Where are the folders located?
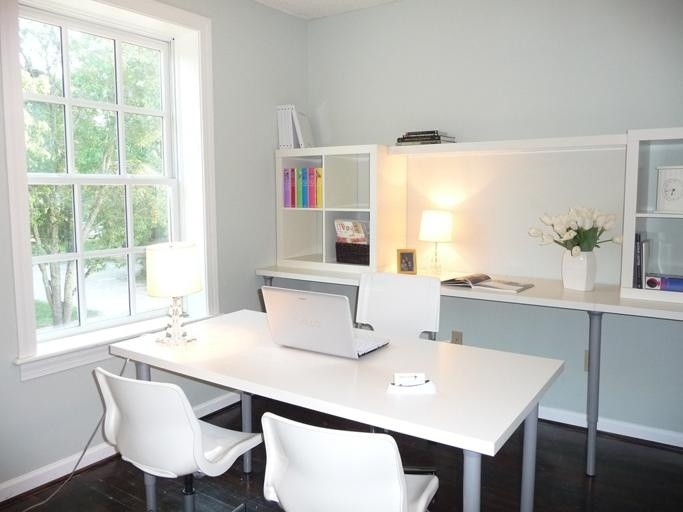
[276,104,316,149]
[282,166,323,208]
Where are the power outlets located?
[451,331,462,344]
[584,350,589,372]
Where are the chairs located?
[260,411,437,511]
[356,270,441,343]
[93,367,261,511]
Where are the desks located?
[110,307,563,512]
[257,267,681,478]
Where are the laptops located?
[260,286,390,359]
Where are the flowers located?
[528,206,624,251]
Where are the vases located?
[561,250,597,292]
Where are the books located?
[633,231,652,291]
[395,129,457,145]
[441,271,536,295]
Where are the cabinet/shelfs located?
[619,129,650,303]
[276,144,405,277]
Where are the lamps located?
[418,209,451,273]
[146,241,206,346]
